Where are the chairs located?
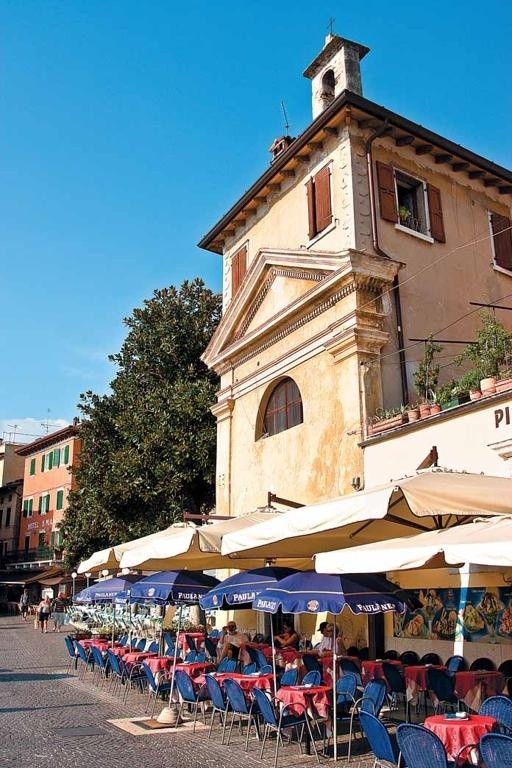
[134,618,143,629]
[111,617,119,628]
[358,647,371,658]
[185,648,196,662]
[84,609,90,620]
[300,654,322,670]
[122,652,158,664]
[137,639,147,652]
[391,721,448,768]
[399,650,419,665]
[425,669,461,714]
[257,663,273,673]
[116,635,127,644]
[279,666,297,686]
[223,678,261,745]
[250,686,325,765]
[149,641,159,653]
[382,649,398,659]
[88,645,106,687]
[88,610,94,620]
[443,656,463,673]
[343,678,386,762]
[105,607,162,621]
[138,664,179,718]
[300,670,322,686]
[420,653,441,666]
[227,658,240,674]
[65,635,91,673]
[250,646,267,667]
[228,641,240,657]
[329,673,361,745]
[64,604,82,616]
[356,709,401,767]
[264,635,274,646]
[106,614,112,626]
[173,669,209,735]
[216,655,228,673]
[86,603,102,609]
[126,620,136,629]
[94,613,99,627]
[165,647,181,658]
[346,643,359,657]
[468,659,495,672]
[129,636,138,647]
[100,616,106,626]
[97,615,102,626]
[74,640,97,683]
[105,649,120,696]
[203,674,241,744]
[186,634,197,650]
[242,662,256,675]
[163,632,175,647]
[383,663,412,717]
[251,633,263,644]
[208,628,218,635]
[360,659,404,687]
[480,734,510,767]
[117,660,147,699]
[205,636,218,657]
[118,618,128,629]
[478,697,512,735]
[195,654,207,662]
[498,660,512,675]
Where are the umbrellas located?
[76,521,203,574]
[118,504,316,572]
[314,522,512,574]
[198,566,299,610]
[251,570,425,614]
[221,465,512,558]
[113,571,222,605]
[72,574,148,604]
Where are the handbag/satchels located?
[34,612,39,630]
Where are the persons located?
[321,623,346,656]
[36,595,52,633]
[19,589,30,620]
[275,622,300,648]
[51,592,67,633]
[316,622,333,656]
[216,621,244,648]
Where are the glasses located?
[229,624,234,629]
[283,624,288,630]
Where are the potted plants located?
[459,369,482,402]
[454,307,512,396]
[435,380,470,410]
[492,360,512,393]
[408,402,419,422]
[414,336,441,418]
[430,391,440,415]
[369,403,405,433]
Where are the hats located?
[318,621,328,633]
